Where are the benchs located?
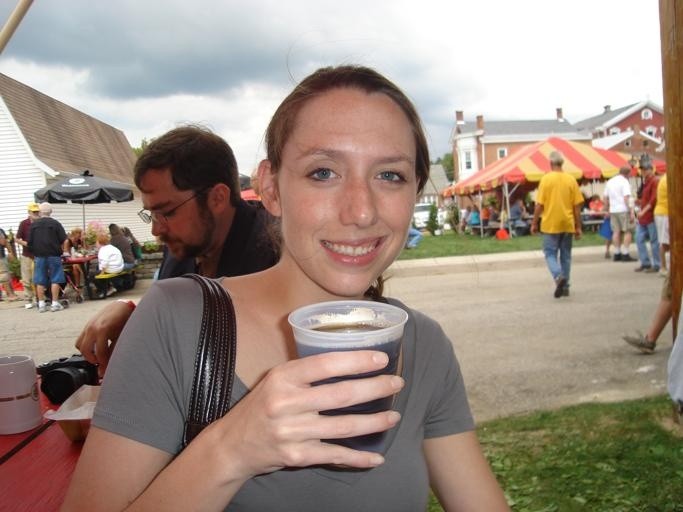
[473,219,527,239]
[94,265,143,298]
[583,219,605,231]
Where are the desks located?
[62,254,97,300]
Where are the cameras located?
[35,350,100,406]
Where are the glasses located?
[138,193,197,225]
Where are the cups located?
[286,298,411,472]
[0,355,43,438]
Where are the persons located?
[621,270,672,353]
[601,165,639,262]
[0,199,145,316]
[597,210,616,260]
[55,63,513,512]
[455,192,646,237]
[528,151,585,298]
[652,172,670,280]
[404,229,423,250]
[71,122,281,377]
[634,161,660,274]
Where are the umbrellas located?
[34,172,134,233]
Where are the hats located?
[638,162,653,170]
[28,204,40,211]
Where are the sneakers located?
[24,303,33,309]
[553,253,670,298]
[39,304,47,313]
[50,303,64,312]
[98,286,117,298]
[622,335,656,354]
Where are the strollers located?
[45,268,84,308]
